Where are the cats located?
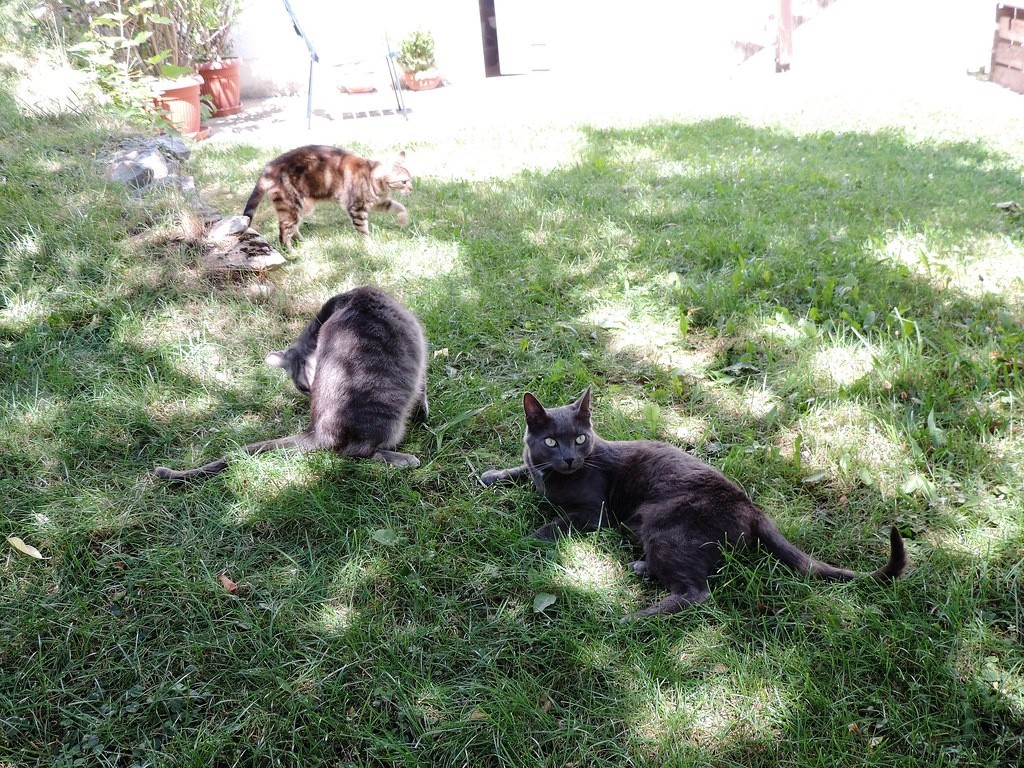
[243,144,413,253]
[480,383,906,624]
[152,286,429,485]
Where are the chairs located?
[284,1,409,129]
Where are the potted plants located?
[395,25,443,91]
[62,0,245,142]
